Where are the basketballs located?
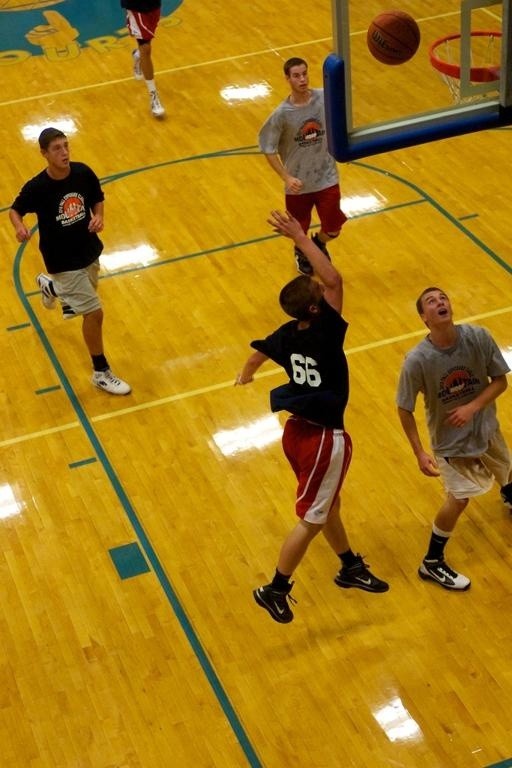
[368,11,419,65]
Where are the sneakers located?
[150,91,164,116]
[92,370,131,395]
[36,273,56,310]
[253,584,293,623]
[418,556,471,591]
[294,232,331,276]
[132,49,143,79]
[335,556,388,593]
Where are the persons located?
[232,211,388,623]
[257,56,348,274]
[395,287,511,593]
[117,0,167,117]
[9,128,132,396]
